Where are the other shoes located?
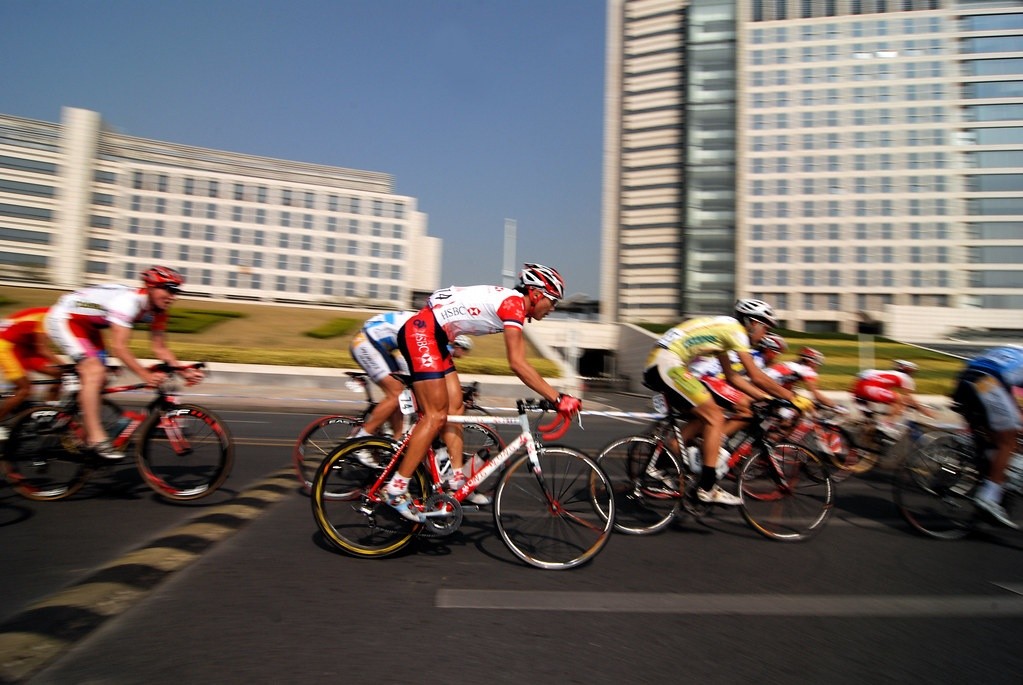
[84,437,125,458]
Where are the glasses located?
[533,286,560,306]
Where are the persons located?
[42,265,203,461]
[643,297,834,506]
[0,305,67,440]
[853,357,936,426]
[380,262,582,521]
[349,311,472,471]
[954,344,1023,531]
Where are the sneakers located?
[450,480,493,505]
[698,485,744,505]
[967,492,1018,527]
[379,488,426,523]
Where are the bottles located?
[715,445,732,470]
[460,448,491,478]
[381,421,396,440]
[109,411,136,439]
[432,440,455,481]
[685,438,702,473]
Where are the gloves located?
[791,394,816,413]
[554,393,583,421]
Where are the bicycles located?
[624,389,945,530]
[0,361,132,469]
[587,380,836,544]
[0,363,237,501]
[293,372,512,508]
[311,372,616,571]
[891,403,1023,541]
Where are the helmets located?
[141,264,183,287]
[734,299,777,329]
[894,358,919,372]
[454,335,472,349]
[798,345,824,367]
[519,261,564,300]
[757,330,788,355]
